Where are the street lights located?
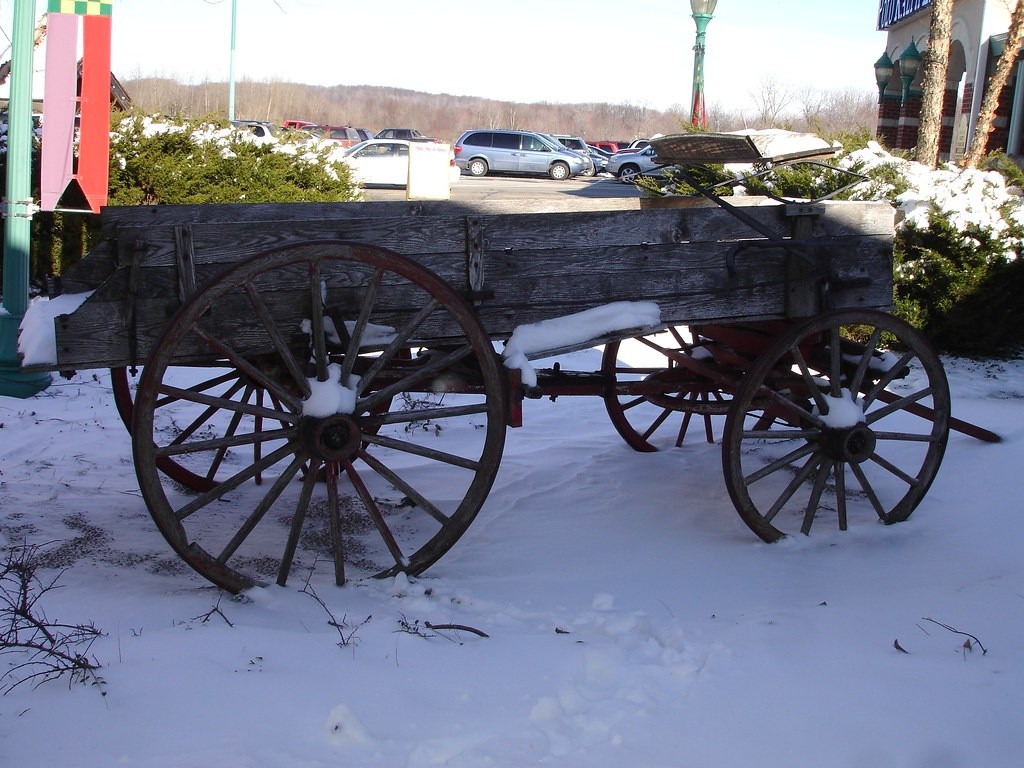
[690,0,717,130]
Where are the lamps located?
[873,45,897,104]
[897,33,925,109]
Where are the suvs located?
[454,128,593,180]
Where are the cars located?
[309,137,460,190]
[531,134,655,178]
[605,145,680,185]
[227,120,441,157]
